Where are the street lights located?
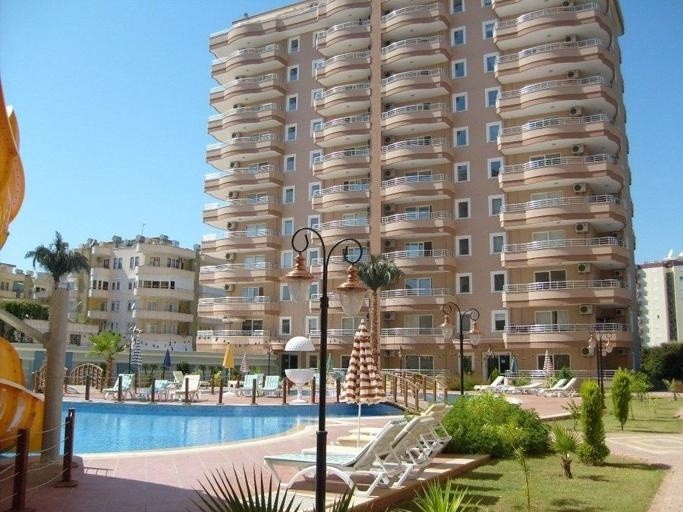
[441,301,481,394]
[588,326,614,394]
[284,226,370,512]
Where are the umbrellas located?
[240,352,250,372]
[130,335,142,393]
[222,341,234,380]
[543,349,555,388]
[511,356,517,373]
[339,319,387,447]
[163,349,171,379]
[326,353,333,372]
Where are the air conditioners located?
[570,142,595,358]
[382,70,397,359]
[222,74,244,292]
[559,2,582,118]
[613,269,626,357]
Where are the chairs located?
[262,402,457,498]
[101,370,284,404]
[472,374,581,399]
